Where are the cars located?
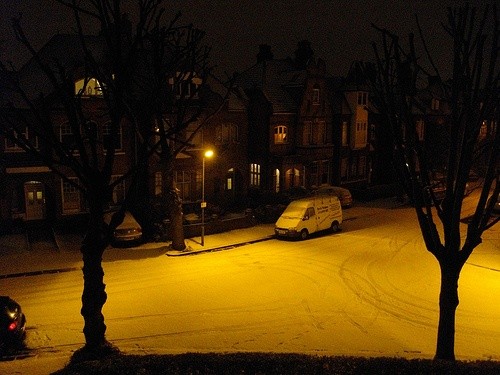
[104,210,144,244]
[317,186,352,207]
[0,295,27,354]
[486,193,500,213]
[406,167,469,206]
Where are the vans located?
[273,193,342,241]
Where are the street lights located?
[201,149,215,247]
